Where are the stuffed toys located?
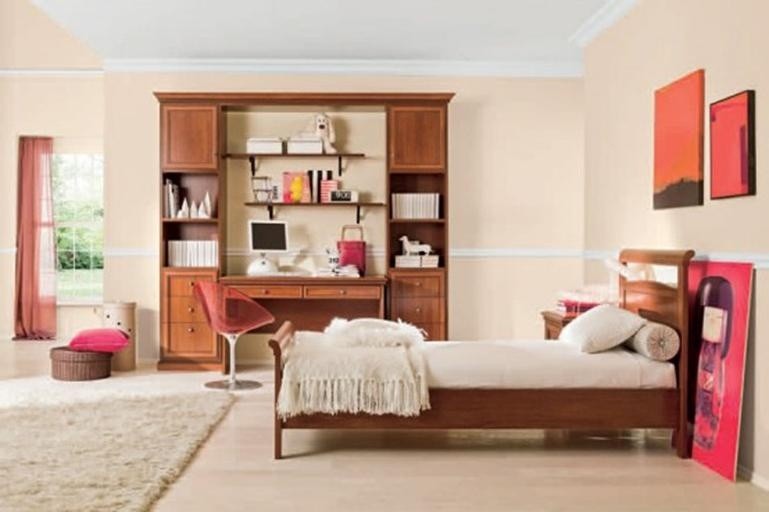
[309,114,339,155]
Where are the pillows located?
[70,328,129,354]
[557,303,647,353]
[622,319,681,361]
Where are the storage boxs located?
[50,347,112,380]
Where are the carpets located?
[1,340,237,512]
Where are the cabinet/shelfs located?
[153,93,456,373]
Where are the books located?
[168,239,218,267]
[395,255,439,266]
[554,298,598,319]
[392,192,440,219]
[164,177,179,219]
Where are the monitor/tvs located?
[248,221,288,274]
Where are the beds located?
[268,248,695,460]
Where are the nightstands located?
[541,309,573,340]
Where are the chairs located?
[191,279,275,393]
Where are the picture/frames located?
[653,69,704,210]
[709,90,756,199]
